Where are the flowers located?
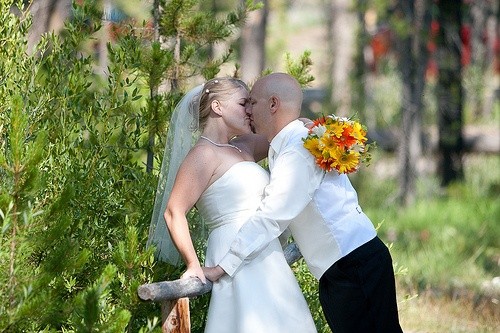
[302,111,376,174]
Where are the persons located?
[163,77,318,332]
[201,72,403,333]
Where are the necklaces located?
[200,135,242,153]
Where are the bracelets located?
[304,122,315,127]
